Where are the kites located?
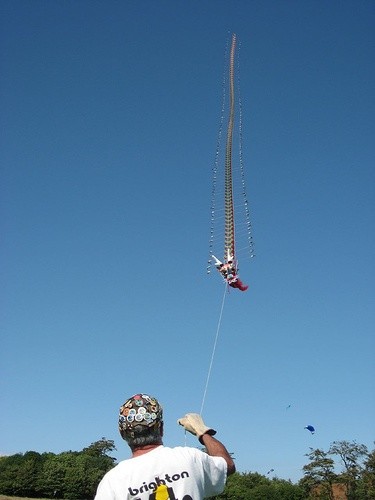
[304,424,316,436]
[206,31,257,291]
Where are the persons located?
[94,393,237,499]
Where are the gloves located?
[179,413,216,444]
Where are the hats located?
[119,393,162,438]
